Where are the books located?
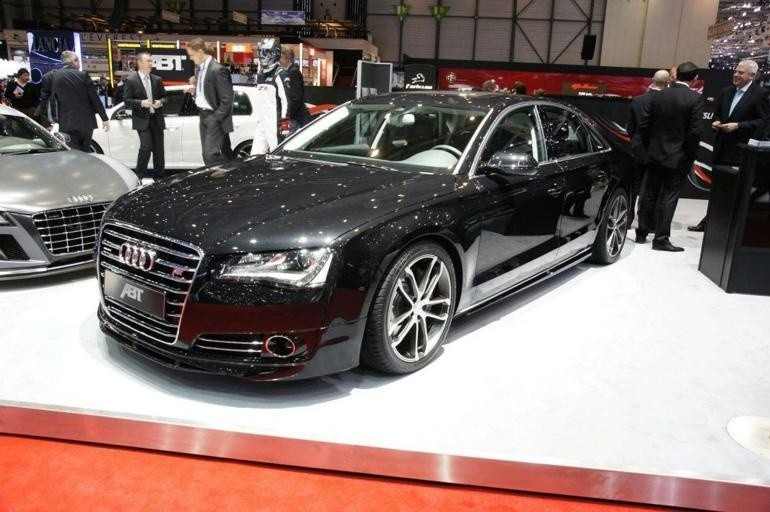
[14,86,24,95]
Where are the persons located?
[627,69,670,233]
[481,79,545,96]
[249,36,291,154]
[635,60,704,252]
[187,38,233,165]
[0,73,126,136]
[687,59,770,232]
[277,46,313,135]
[645,66,678,91]
[123,52,167,185]
[4,68,39,120]
[40,50,111,153]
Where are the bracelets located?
[736,122,738,130]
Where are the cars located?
[47,83,267,171]
[0,104,142,283]
[95,87,634,383]
[279,102,337,135]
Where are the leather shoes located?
[687,221,704,232]
[635,233,646,243]
[652,237,684,252]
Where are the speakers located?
[581,35,596,60]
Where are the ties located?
[199,65,204,94]
[143,76,152,100]
[729,90,743,117]
